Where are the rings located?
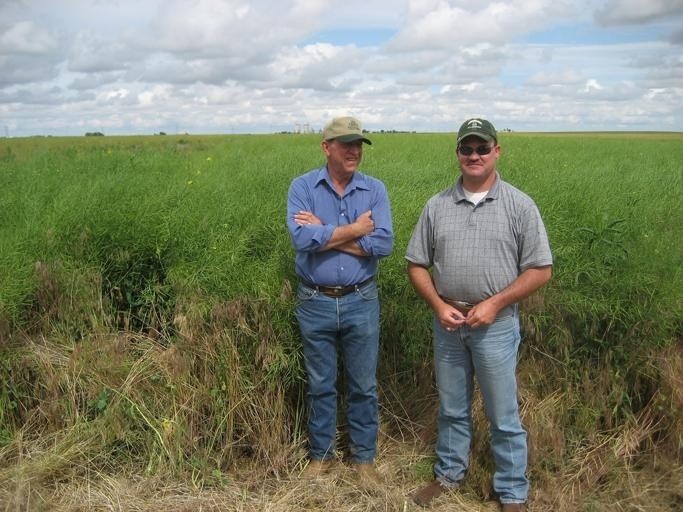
[307,223,311,225]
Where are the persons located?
[284,115,395,485]
[404,117,554,512]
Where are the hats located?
[456,118,498,146]
[323,115,372,148]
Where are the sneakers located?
[352,462,372,481]
[301,458,332,479]
[502,501,527,512]
[414,479,466,507]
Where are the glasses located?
[458,146,496,155]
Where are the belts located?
[302,275,375,297]
[441,297,476,315]
